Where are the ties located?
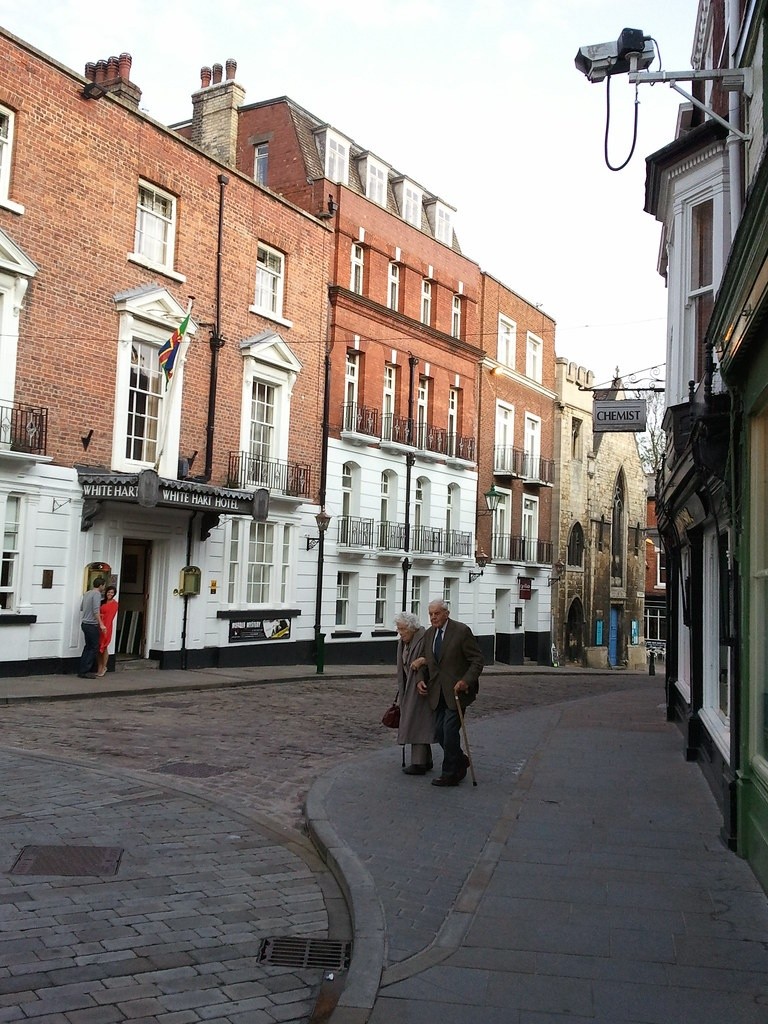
[434,629,443,661]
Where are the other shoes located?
[432,775,458,787]
[403,761,433,776]
[456,754,470,780]
[78,673,96,679]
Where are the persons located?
[93,586,119,676]
[393,611,438,775]
[415,599,485,786]
[78,578,106,679]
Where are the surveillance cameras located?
[574,35,655,82]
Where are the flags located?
[158,312,189,392]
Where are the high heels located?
[95,667,107,676]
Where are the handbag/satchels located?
[382,691,400,729]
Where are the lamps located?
[469,546,489,583]
[547,559,565,587]
[476,481,503,517]
